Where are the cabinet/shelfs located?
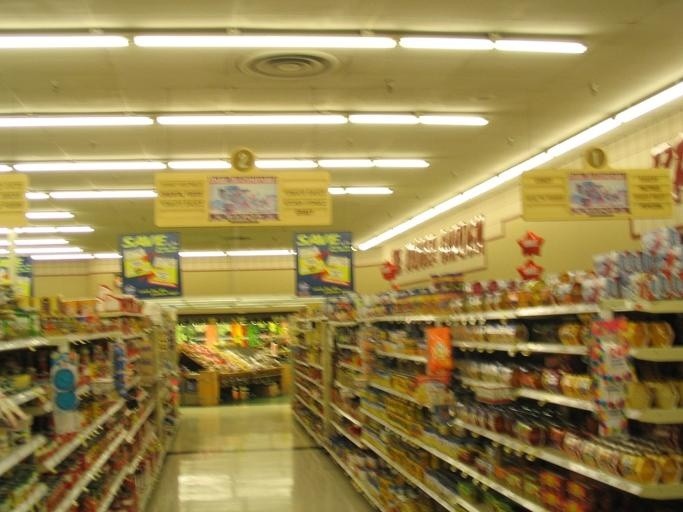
[291,296,683,512]
[0,325,180,512]
